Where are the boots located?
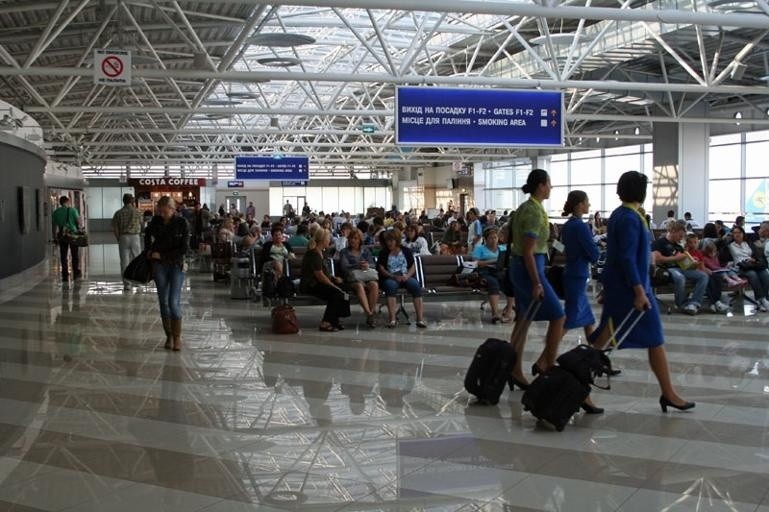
[163,318,174,349]
[172,319,182,351]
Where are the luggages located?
[522,304,649,432]
[464,296,544,404]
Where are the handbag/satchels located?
[57,226,88,247]
[449,272,479,286]
[556,344,617,384]
[348,267,378,282]
[271,305,297,334]
[498,266,515,295]
[546,266,567,300]
[123,250,153,284]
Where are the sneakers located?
[417,320,427,327]
[729,279,747,289]
[712,302,734,313]
[683,304,697,314]
[759,299,769,311]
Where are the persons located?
[262,332,417,427]
[52,197,81,281]
[114,291,142,376]
[113,194,144,290]
[507,390,694,512]
[532,191,624,377]
[144,196,191,351]
[507,170,567,390]
[578,171,695,413]
[138,353,194,482]
[681,311,769,384]
[55,282,86,362]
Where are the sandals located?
[319,324,337,332]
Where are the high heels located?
[508,374,530,391]
[532,363,545,375]
[597,367,621,376]
[660,395,695,413]
[580,401,603,414]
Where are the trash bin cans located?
[230,257,253,299]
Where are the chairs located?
[228,205,763,309]
